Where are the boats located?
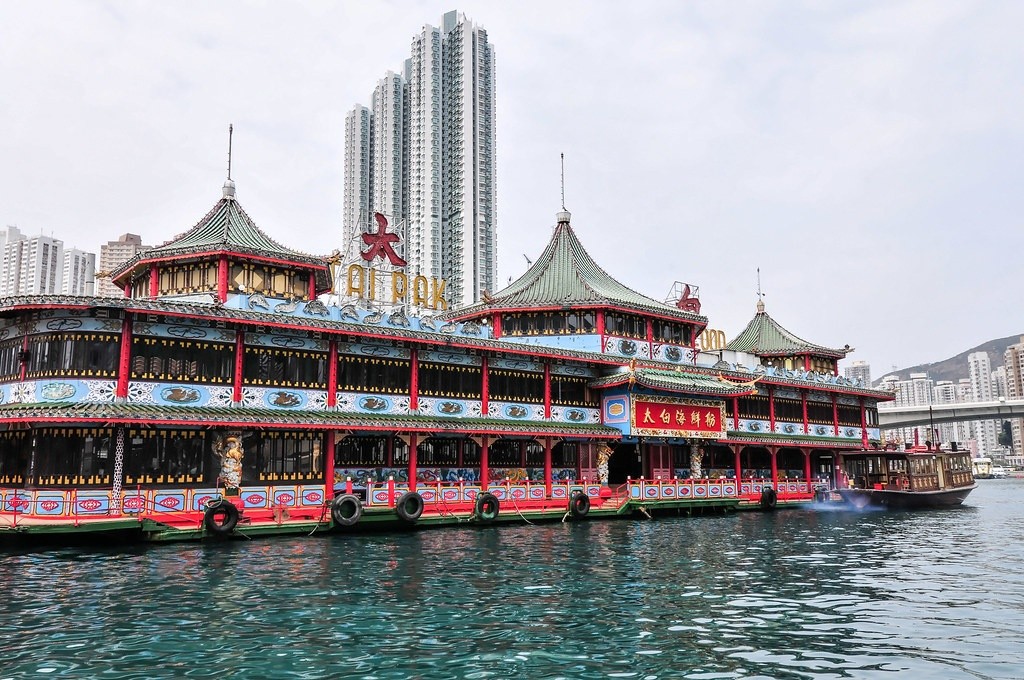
[992,465,1006,479]
[838,439,978,512]
[970,458,994,480]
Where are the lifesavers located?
[569,491,592,519]
[394,490,426,523]
[469,491,502,523]
[761,488,779,509]
[333,492,365,528]
[204,497,240,536]
